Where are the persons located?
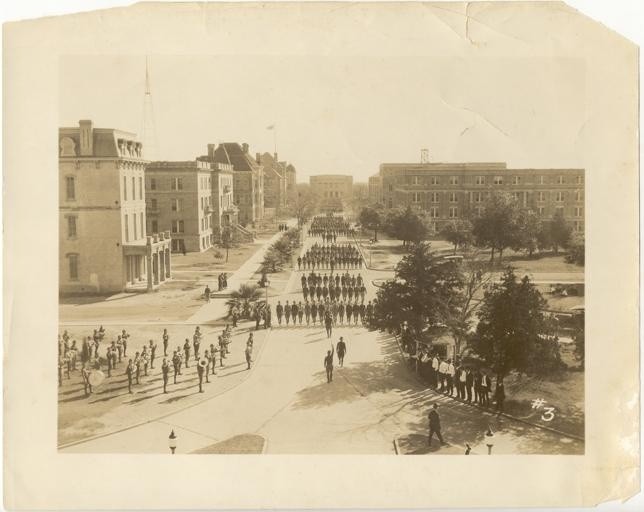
[324,345,334,383]
[205,218,377,326]
[550,284,578,296]
[403,326,504,412]
[336,337,346,366]
[324,310,332,337]
[59,324,253,396]
[428,403,447,446]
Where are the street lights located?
[166,428,176,455]
[369,239,373,266]
[279,232,284,271]
[295,209,300,228]
[485,424,494,455]
[263,278,270,306]
